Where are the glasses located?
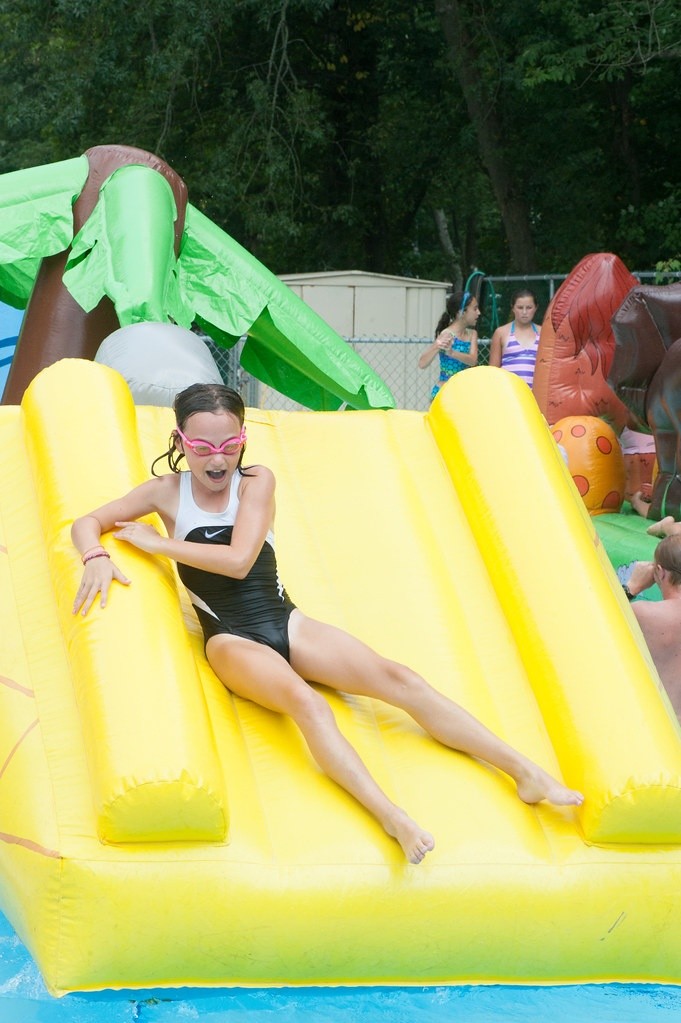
[460,292,470,317]
[177,424,247,457]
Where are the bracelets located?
[83,551,110,565]
[81,545,105,561]
[622,585,634,600]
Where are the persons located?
[69,383,586,865]
[418,291,481,404]
[623,490,681,723]
[487,287,543,390]
